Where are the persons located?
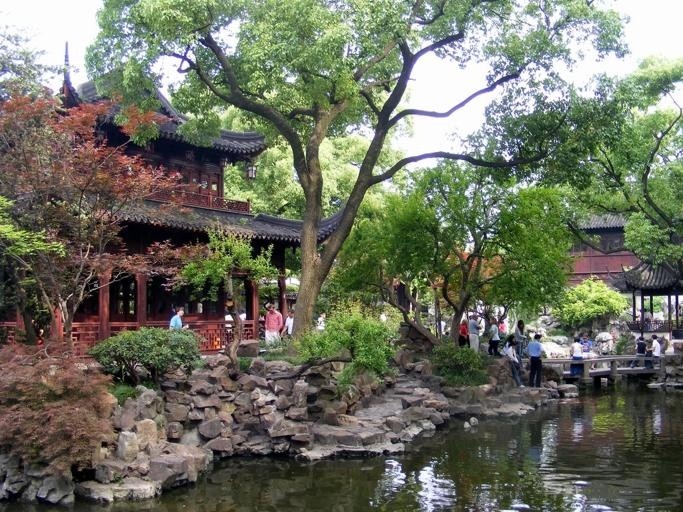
[169,304,190,332]
[436,317,447,335]
[264,302,283,350]
[645,335,660,368]
[281,307,295,336]
[458,312,545,387]
[630,336,648,367]
[643,308,652,321]
[581,333,597,370]
[315,310,327,330]
[239,310,246,326]
[569,336,584,376]
[224,311,234,332]
